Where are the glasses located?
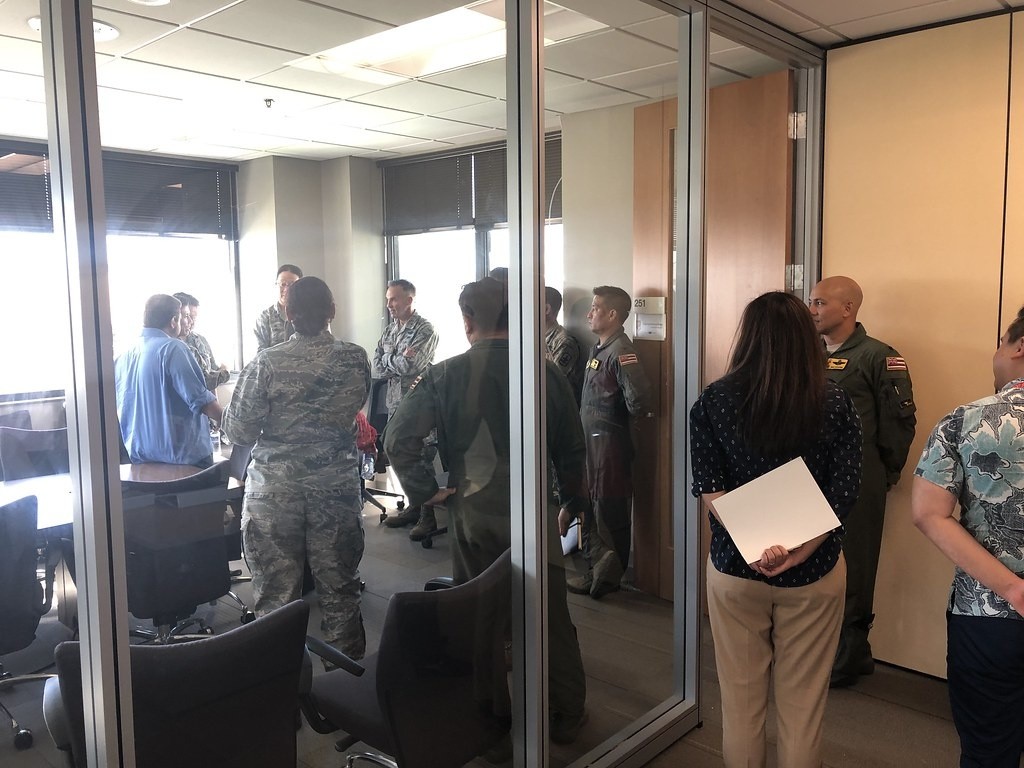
[275,278,293,288]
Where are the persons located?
[544,286,581,390]
[252,264,304,355]
[689,292,866,768]
[173,292,230,392]
[567,286,650,599]
[219,276,371,673]
[114,294,224,470]
[371,279,439,542]
[912,306,1024,768]
[810,276,918,689]
[378,276,589,746]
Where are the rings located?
[769,563,775,566]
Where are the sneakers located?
[589,550,618,599]
[567,574,593,595]
[826,638,874,689]
[383,505,420,528]
[409,509,437,541]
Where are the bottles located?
[209,426,222,457]
[360,449,375,480]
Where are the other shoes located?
[551,707,588,743]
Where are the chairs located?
[0,427,69,615]
[0,495,63,752]
[227,436,259,584]
[357,377,405,521]
[48,459,255,645]
[299,546,512,768]
[42,600,310,768]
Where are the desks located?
[0,463,244,683]
